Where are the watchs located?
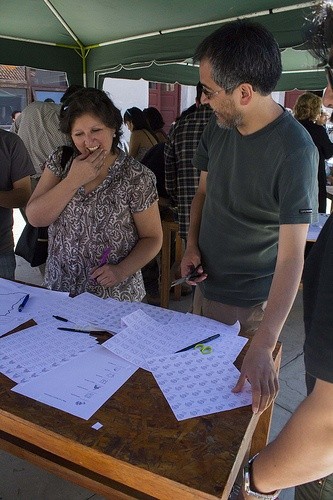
[243,454,280,500]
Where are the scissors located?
[191,344,212,358]
[170,264,206,287]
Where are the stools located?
[160,221,184,309]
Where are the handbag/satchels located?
[12,146,73,267]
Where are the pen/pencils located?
[17,293,29,312]
[53,316,68,323]
[175,334,220,354]
[57,327,91,335]
[100,248,111,266]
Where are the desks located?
[0,280,284,500]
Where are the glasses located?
[201,82,236,100]
[324,67,333,89]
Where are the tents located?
[0,0,333,90]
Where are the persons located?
[0,128,37,281]
[10,86,78,223]
[237,2,333,500]
[293,90,332,213]
[122,82,214,295]
[25,87,163,304]
[180,19,319,413]
